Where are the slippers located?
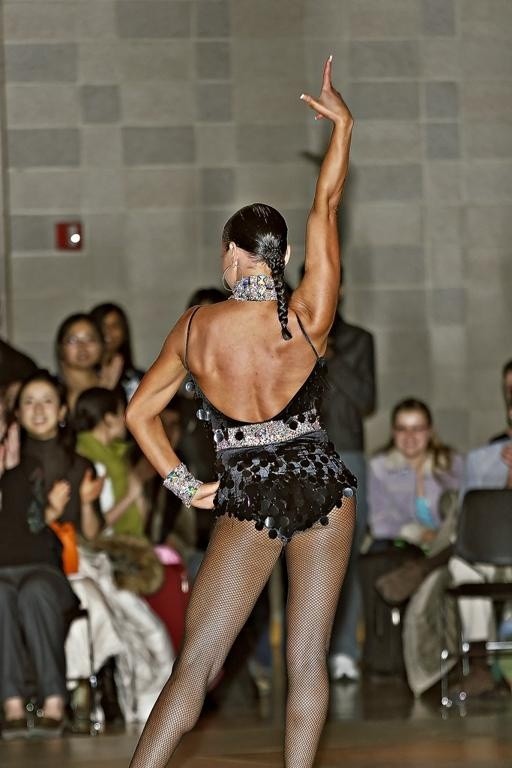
[3,707,29,740]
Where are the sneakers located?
[330,652,360,684]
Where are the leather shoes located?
[33,712,70,736]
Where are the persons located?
[298,258,380,684]
[0,286,228,731]
[352,360,512,694]
[119,52,354,768]
